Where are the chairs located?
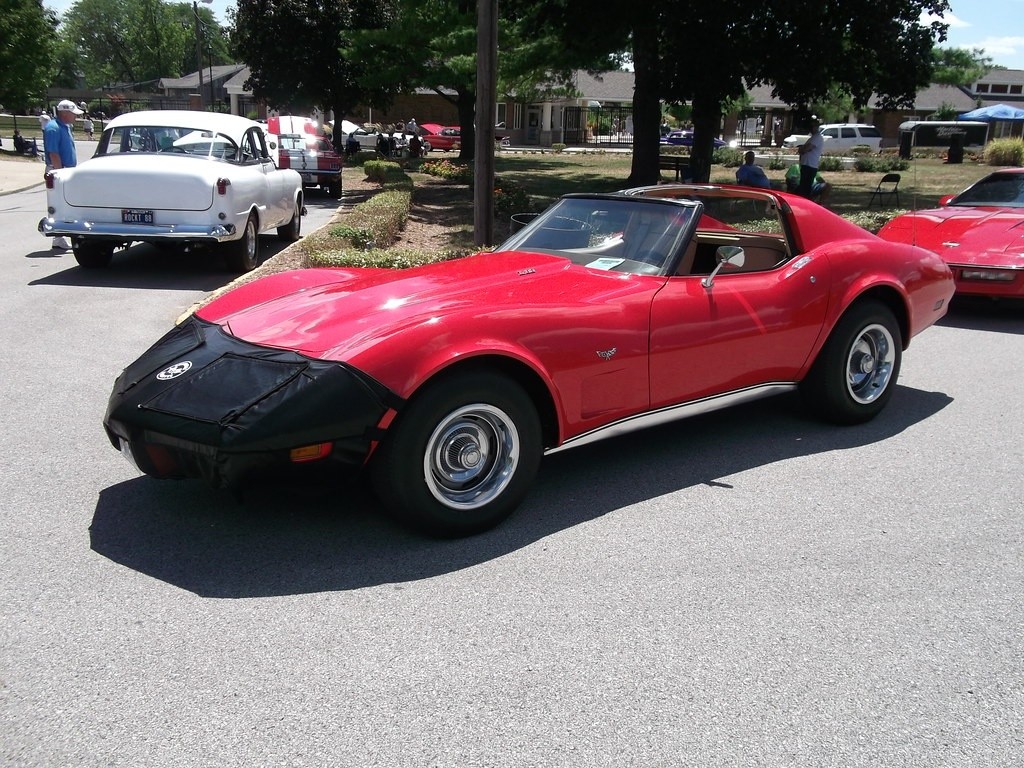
[33,138,45,161]
[335,142,361,163]
[727,237,786,272]
[867,174,901,209]
[634,224,699,275]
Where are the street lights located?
[206,44,215,112]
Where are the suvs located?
[781,122,883,157]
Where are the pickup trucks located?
[660,131,727,150]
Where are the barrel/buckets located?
[509,213,592,250]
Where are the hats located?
[42,111,46,114]
[57,98,84,114]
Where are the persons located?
[611,116,690,135]
[736,119,833,207]
[344,118,428,159]
[14,100,98,250]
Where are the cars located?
[328,120,424,157]
[38,109,343,272]
[419,123,510,152]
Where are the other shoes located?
[52,238,72,249]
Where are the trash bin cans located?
[761,134,772,147]
[510,212,591,249]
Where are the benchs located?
[659,156,689,181]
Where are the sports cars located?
[876,167,1024,298]
[103,182,956,541]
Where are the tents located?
[958,103,1024,149]
